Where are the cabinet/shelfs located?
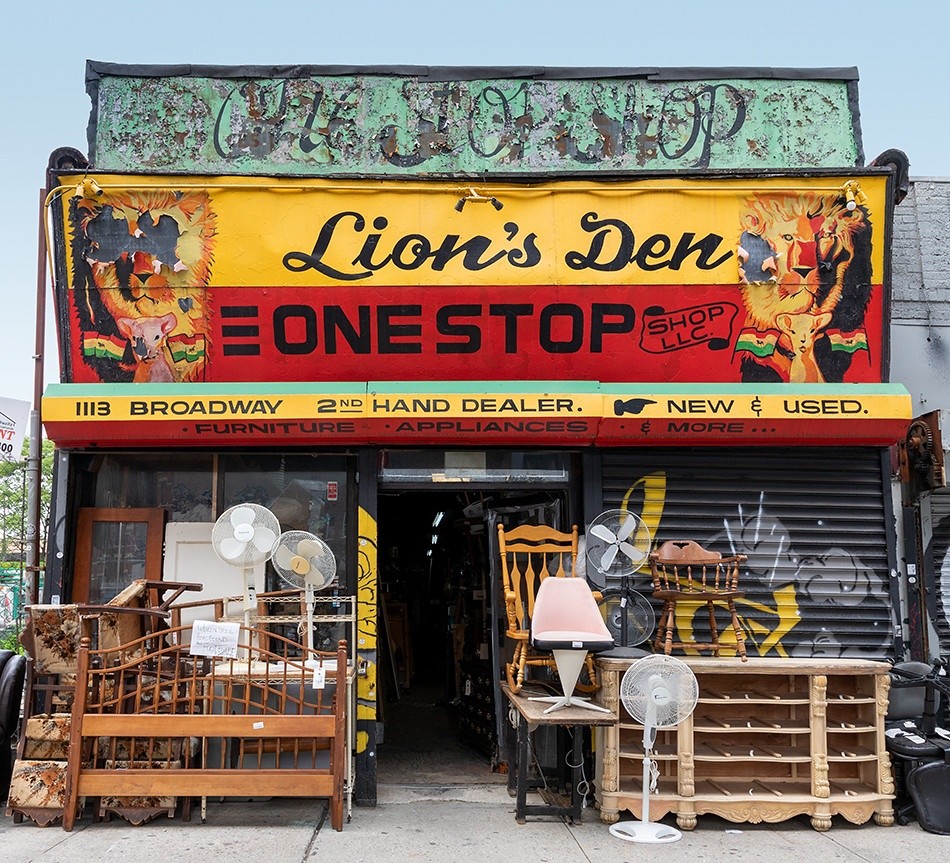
[598,655,896,832]
[385,602,413,693]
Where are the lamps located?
[842,182,867,210]
[74,180,104,200]
[454,190,504,212]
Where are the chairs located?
[646,539,749,665]
[497,522,603,695]
[889,658,950,838]
[528,576,616,715]
[5,578,210,826]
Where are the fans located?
[608,654,698,845]
[585,508,653,658]
[212,502,281,674]
[269,530,336,667]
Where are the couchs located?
[0,650,27,805]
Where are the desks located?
[200,658,356,822]
[501,681,619,823]
